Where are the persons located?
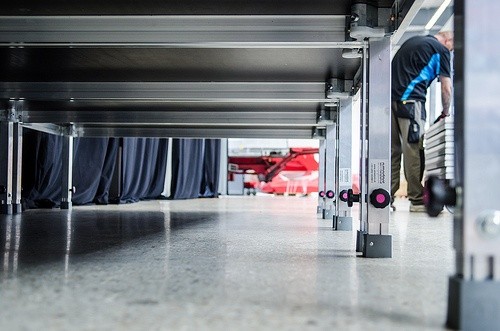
[392,28,457,212]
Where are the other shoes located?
[410,202,427,212]
